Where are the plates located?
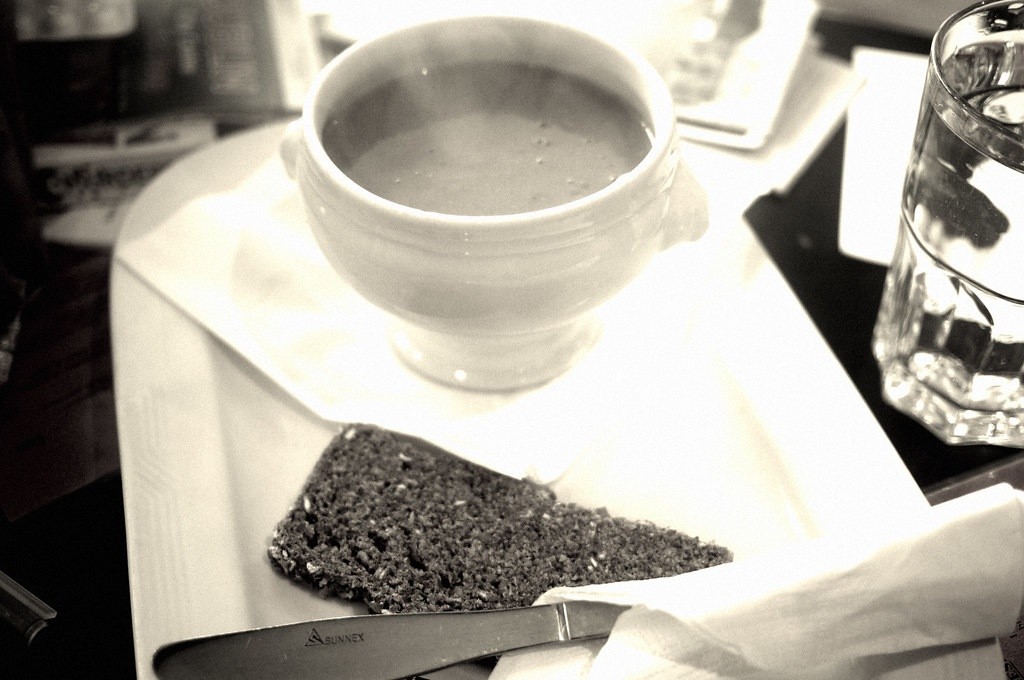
[110,0,1024,680]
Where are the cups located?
[873,0,1024,445]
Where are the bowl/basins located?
[280,17,709,395]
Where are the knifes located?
[151,601,631,680]
